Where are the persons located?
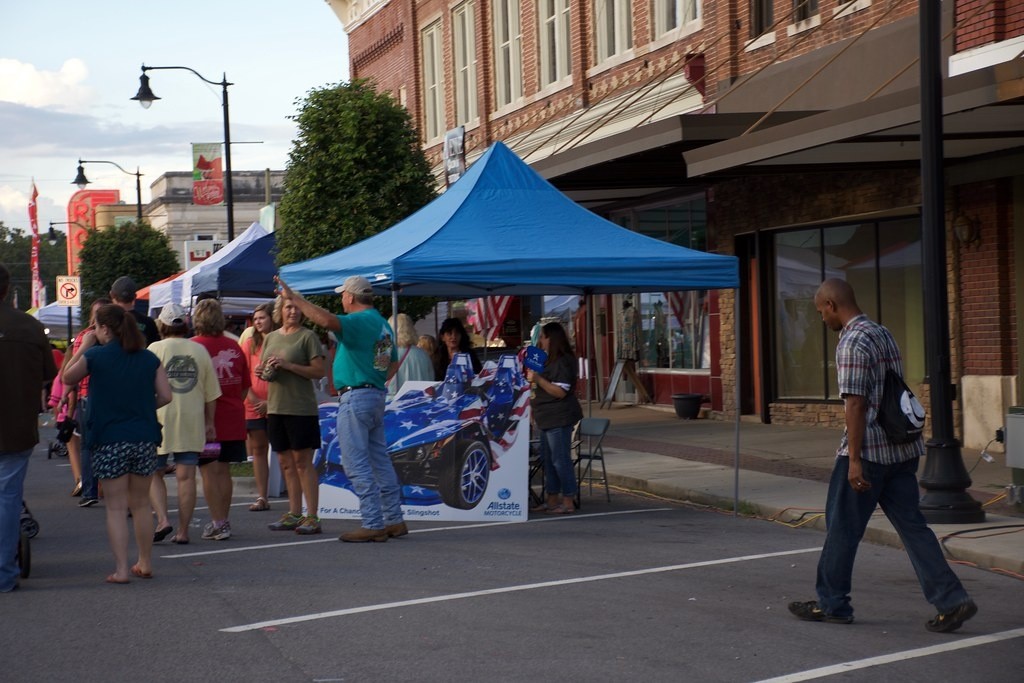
[255,290,329,536]
[44,276,276,542]
[787,276,979,632]
[270,272,411,544]
[59,303,172,586]
[0,265,62,594]
[383,307,471,401]
[522,320,583,516]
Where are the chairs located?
[527,416,611,511]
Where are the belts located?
[339,383,379,394]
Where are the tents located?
[21,221,281,349]
[272,138,742,518]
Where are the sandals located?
[249,496,270,511]
[530,502,559,514]
[546,503,576,516]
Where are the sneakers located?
[387,522,409,538]
[925,600,978,632]
[295,514,321,534]
[788,596,855,625]
[269,512,304,530]
[339,525,388,543]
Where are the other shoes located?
[71,479,84,497]
[200,521,232,540]
[77,498,99,507]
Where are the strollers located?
[47,437,69,460]
[14,500,40,579]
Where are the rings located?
[858,482,863,487]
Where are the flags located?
[192,140,225,206]
[473,296,512,340]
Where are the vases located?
[670,393,704,419]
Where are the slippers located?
[153,525,174,542]
[129,563,154,579]
[106,572,130,584]
[170,533,189,544]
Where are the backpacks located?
[855,328,926,444]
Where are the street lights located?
[129,63,236,247]
[70,157,145,226]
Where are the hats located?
[158,301,186,327]
[111,275,137,297]
[335,276,374,296]
